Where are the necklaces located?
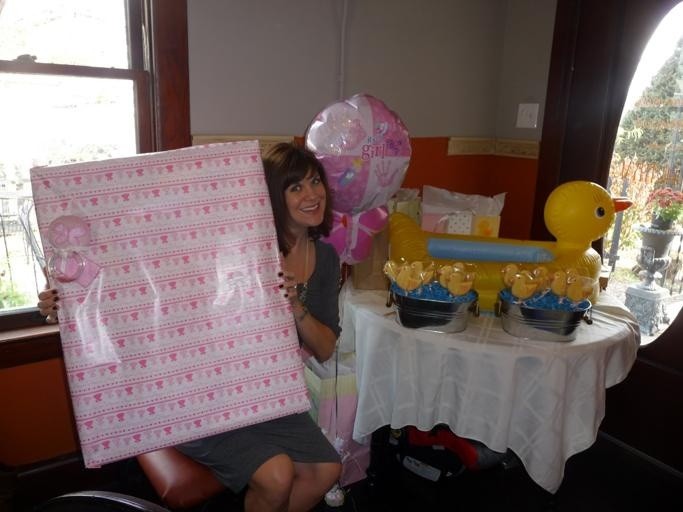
[276,231,310,302]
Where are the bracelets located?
[296,304,310,321]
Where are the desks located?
[338,277,640,486]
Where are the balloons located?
[308,95,410,262]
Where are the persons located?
[37,141,344,512]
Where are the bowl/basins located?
[389,280,479,335]
[497,287,592,341]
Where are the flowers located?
[645,184,683,222]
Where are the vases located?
[651,212,673,230]
